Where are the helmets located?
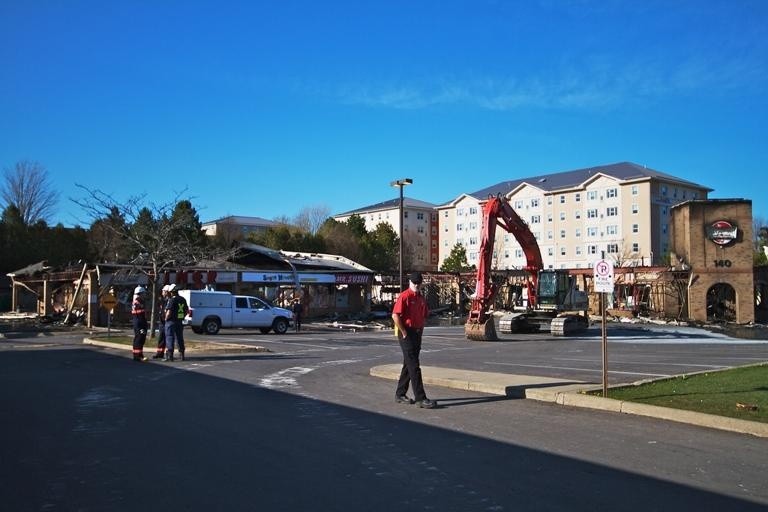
[134,286,146,294]
[161,283,177,292]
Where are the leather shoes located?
[415,398,437,409]
[394,394,413,404]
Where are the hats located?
[409,273,423,283]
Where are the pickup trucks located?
[179,295,295,335]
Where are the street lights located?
[389,178,413,294]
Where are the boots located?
[132,350,187,363]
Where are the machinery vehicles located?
[462,192,589,344]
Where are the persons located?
[290,298,304,333]
[391,270,439,409]
[152,284,175,359]
[161,283,190,362]
[130,285,149,362]
